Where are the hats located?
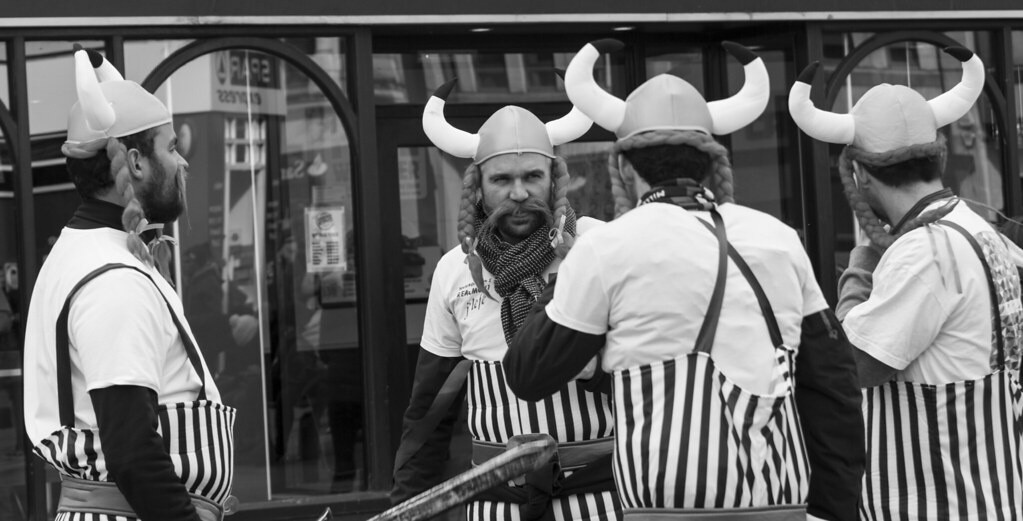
[564,39,770,218]
[60,43,176,286]
[789,46,986,248]
[421,68,593,303]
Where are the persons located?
[24,43,235,521]
[391,68,624,521]
[185,162,403,485]
[787,46,1023,521]
[504,38,864,521]
